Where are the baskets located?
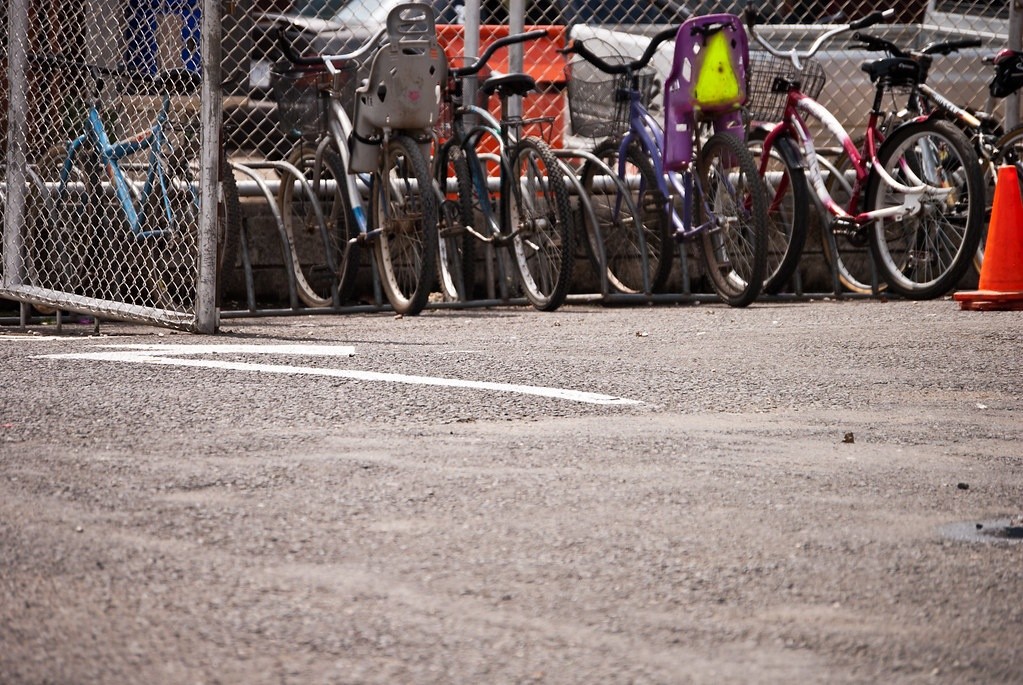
[269,58,360,134]
[437,56,491,123]
[564,55,657,137]
[745,53,826,122]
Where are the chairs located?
[348,3,449,176]
[664,12,750,172]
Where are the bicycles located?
[268,2,1023,315]
[28,55,241,310]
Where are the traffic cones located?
[952,165,1023,310]
